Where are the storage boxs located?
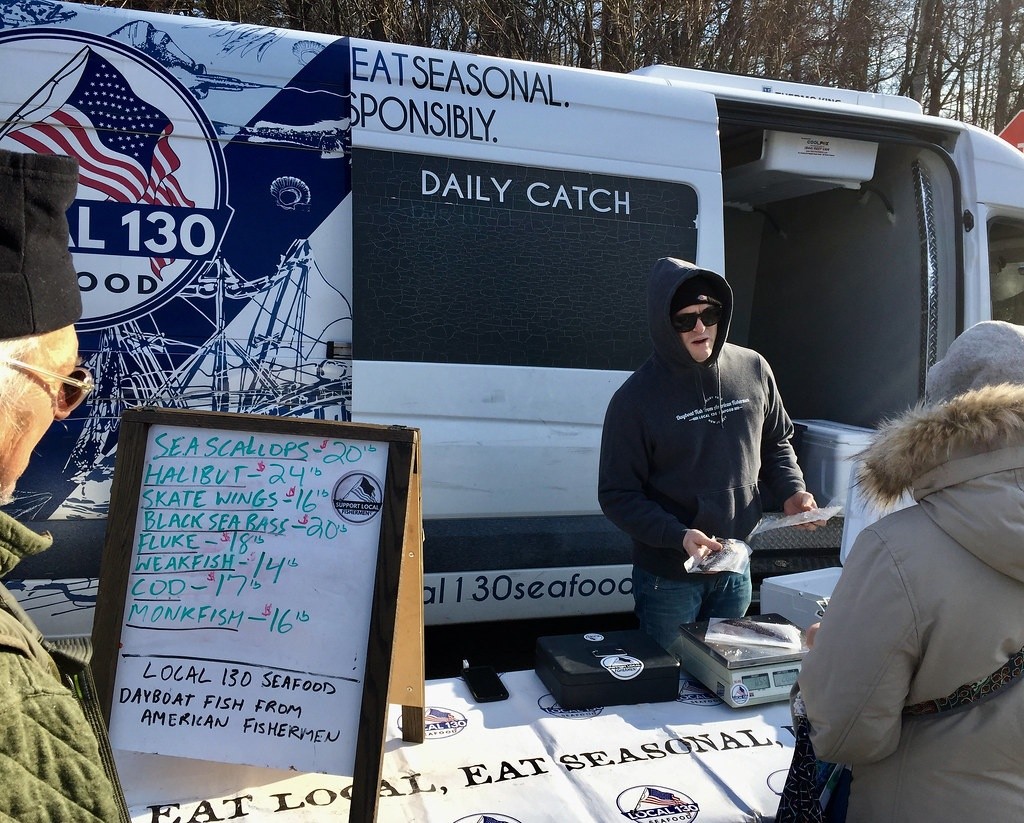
[534,629,681,712]
[760,461,919,632]
[791,418,879,515]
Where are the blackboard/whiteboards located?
[81,406,418,777]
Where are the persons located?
[1,149,145,823]
[793,320,1024,823]
[595,256,832,664]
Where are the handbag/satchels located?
[774,691,826,823]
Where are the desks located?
[115,670,798,823]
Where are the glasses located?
[1,356,95,413]
[671,304,724,333]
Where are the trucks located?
[0,0,1024,680]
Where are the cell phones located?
[462,666,509,703]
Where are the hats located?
[670,277,724,318]
[0,149,83,340]
